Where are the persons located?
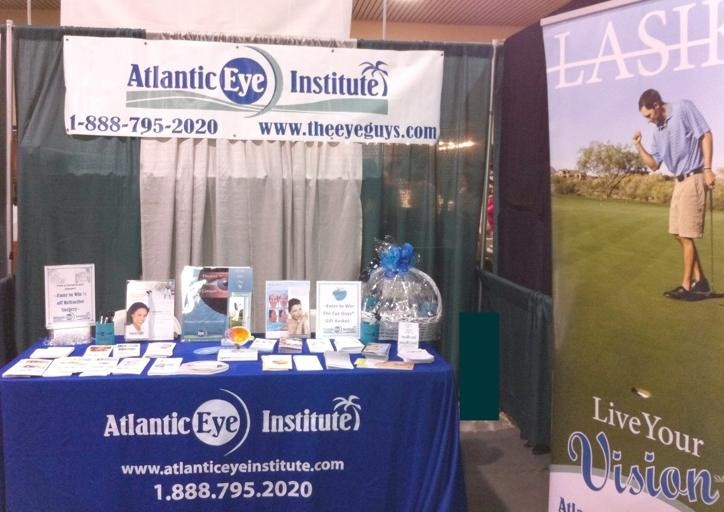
[632,88,717,301]
[286,298,311,339]
[269,295,288,323]
[125,302,149,339]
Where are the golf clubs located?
[706,189,716,297]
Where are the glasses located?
[204,278,228,293]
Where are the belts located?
[678,167,702,182]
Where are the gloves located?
[182,280,207,313]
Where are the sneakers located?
[689,279,711,295]
[663,286,696,301]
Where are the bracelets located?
[703,167,711,169]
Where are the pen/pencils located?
[98,311,116,324]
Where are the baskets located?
[362,264,442,343]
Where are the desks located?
[0,328,454,512]
